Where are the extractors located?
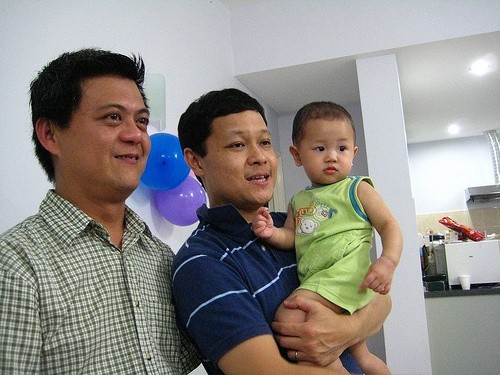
[467,185,499,203]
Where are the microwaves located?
[432,239,499,289]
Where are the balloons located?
[156,175,207,226]
[141,133,192,190]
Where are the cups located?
[459,275,472,290]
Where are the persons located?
[1,49,202,375]
[253,100,403,374]
[171,89,392,375]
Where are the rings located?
[295,351,298,362]
